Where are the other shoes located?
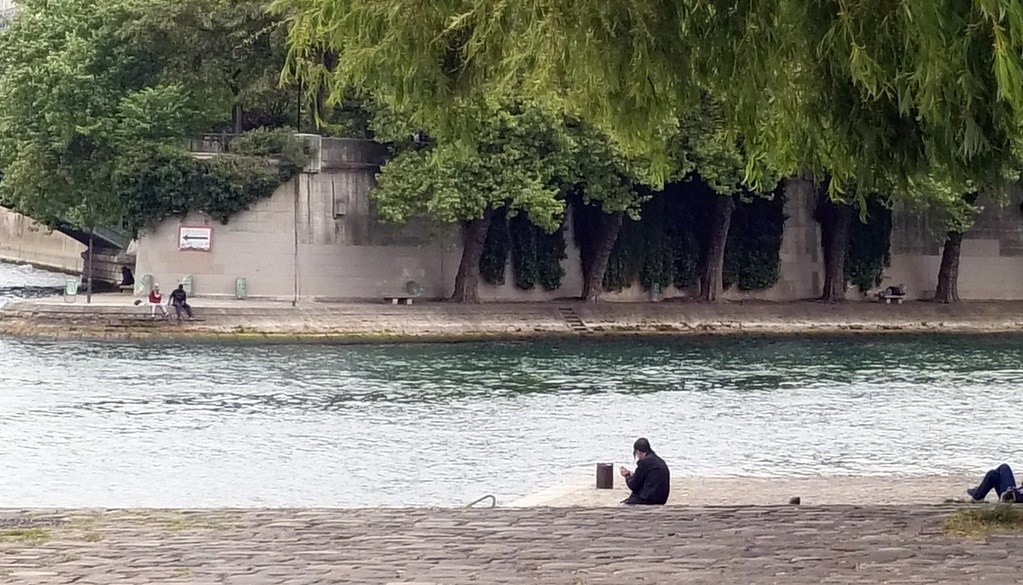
[967,489,980,499]
[151,314,155,319]
[164,312,169,317]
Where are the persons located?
[967,463,1023,503]
[168,284,194,320]
[620,438,670,505]
[148,283,170,318]
[120,267,134,292]
